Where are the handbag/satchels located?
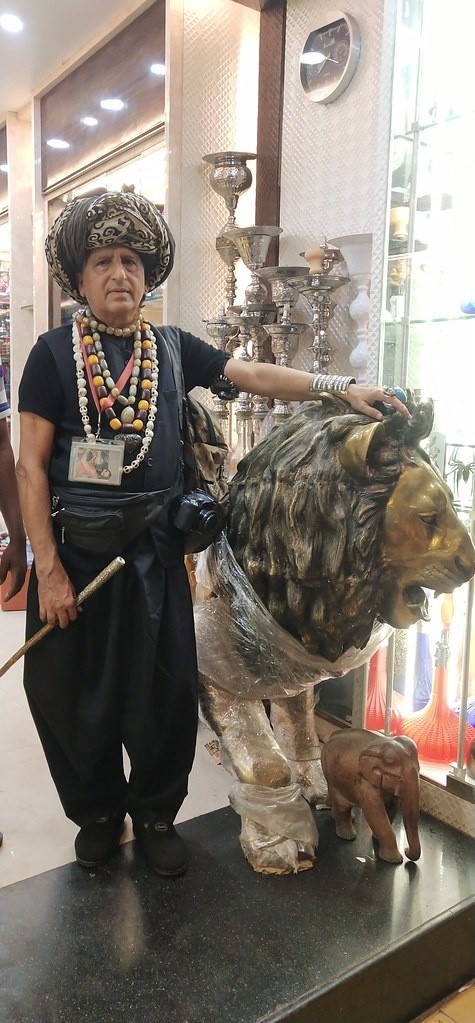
[49,482,185,552]
[179,393,232,553]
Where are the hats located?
[44,187,176,306]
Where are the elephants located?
[321,728,422,864]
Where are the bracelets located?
[311,374,356,393]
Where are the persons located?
[76,448,112,480]
[14,185,412,878]
[0,357,27,600]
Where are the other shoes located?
[74,807,127,866]
[132,818,188,875]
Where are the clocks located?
[298,10,360,104]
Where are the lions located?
[185,388,475,877]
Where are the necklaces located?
[71,308,160,472]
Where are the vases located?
[364,640,410,731]
[398,630,475,762]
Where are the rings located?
[382,388,395,396]
